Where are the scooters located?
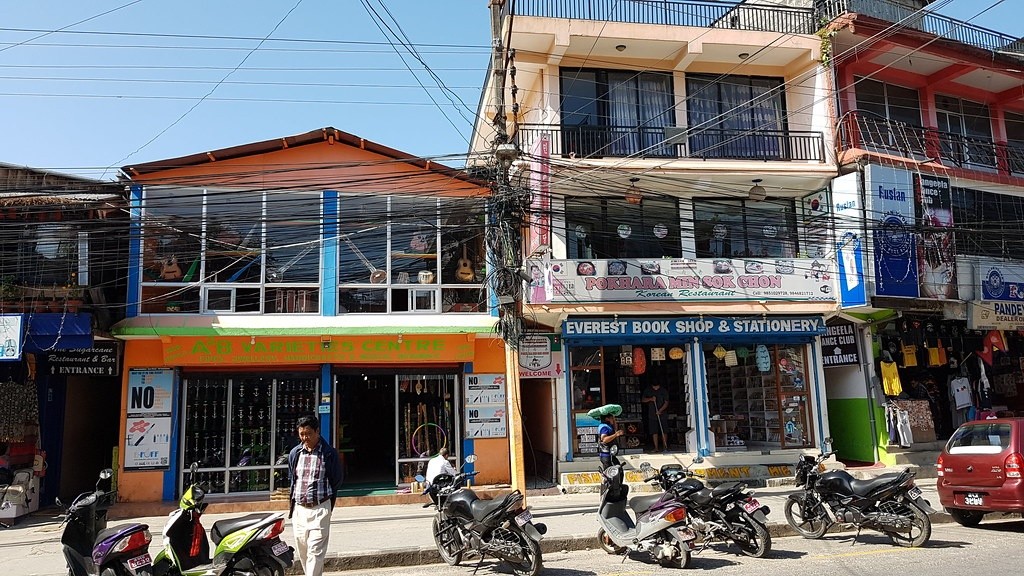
[415,454,547,576]
[56,468,154,576]
[640,456,771,558]
[233,445,290,489]
[596,445,696,569]
[152,461,295,576]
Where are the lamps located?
[616,44,627,51]
[740,53,749,59]
[748,179,766,201]
[626,177,643,204]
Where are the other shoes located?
[663,449,670,453]
[651,449,659,453]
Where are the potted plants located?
[0,274,80,313]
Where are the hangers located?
[882,351,894,363]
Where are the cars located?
[937,418,1024,527]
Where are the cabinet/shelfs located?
[703,349,806,444]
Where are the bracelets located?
[615,432,619,436]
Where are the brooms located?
[653,396,672,454]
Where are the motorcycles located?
[188,447,231,488]
[784,437,937,547]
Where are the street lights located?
[490,143,526,510]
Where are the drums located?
[277,289,312,312]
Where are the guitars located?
[409,223,429,252]
[454,237,475,282]
[160,255,182,280]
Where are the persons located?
[417,444,437,477]
[911,376,940,440]
[598,414,625,501]
[919,371,948,433]
[426,447,458,502]
[641,376,670,451]
[574,379,587,411]
[288,415,343,576]
